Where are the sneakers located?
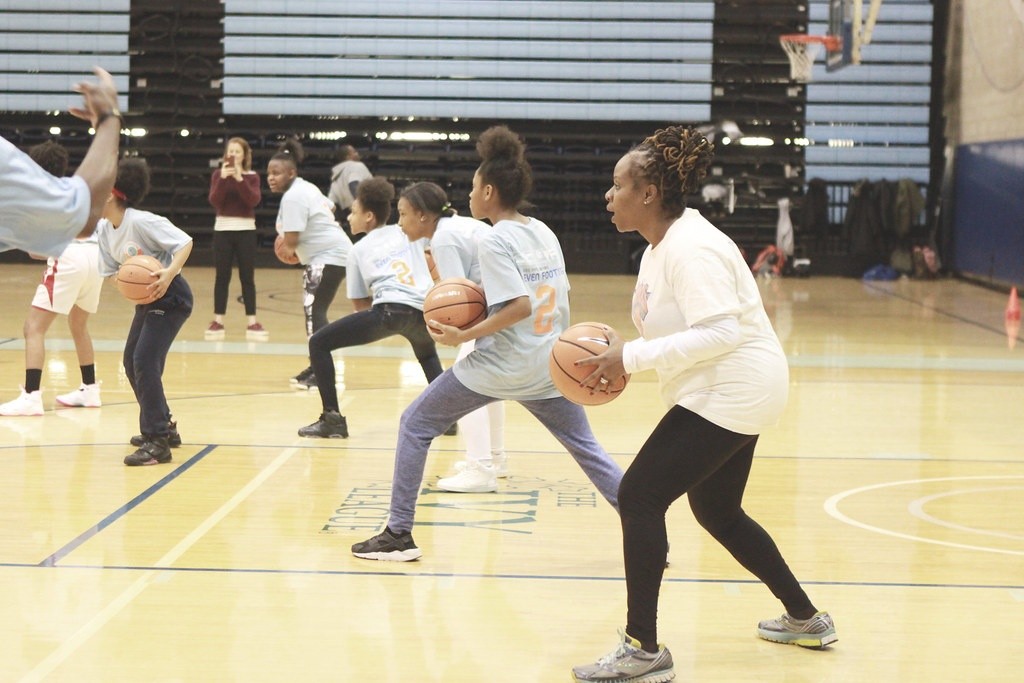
[436,468,497,493]
[455,459,508,478]
[56,381,102,406]
[130,420,182,448]
[756,611,838,647]
[0,389,44,417]
[246,322,269,335]
[572,627,675,683]
[205,321,225,333]
[57,407,102,426]
[1,416,42,439]
[290,366,319,390]
[351,525,423,561]
[298,411,348,439]
[124,437,173,465]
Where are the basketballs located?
[425,250,440,282]
[274,232,301,265]
[351,293,379,313]
[116,253,168,305]
[549,320,632,406]
[425,275,491,342]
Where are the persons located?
[568,125,839,683]
[210,124,669,568]
[0,65,193,465]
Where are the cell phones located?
[224,154,235,167]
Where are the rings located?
[601,377,609,385]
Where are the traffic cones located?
[1005,286,1020,321]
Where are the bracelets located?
[96,109,126,132]
